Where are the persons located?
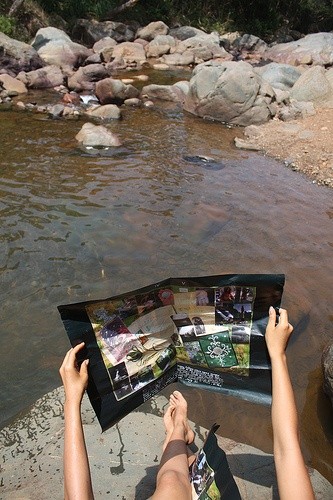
[59,307,317,500]
[219,287,235,310]
